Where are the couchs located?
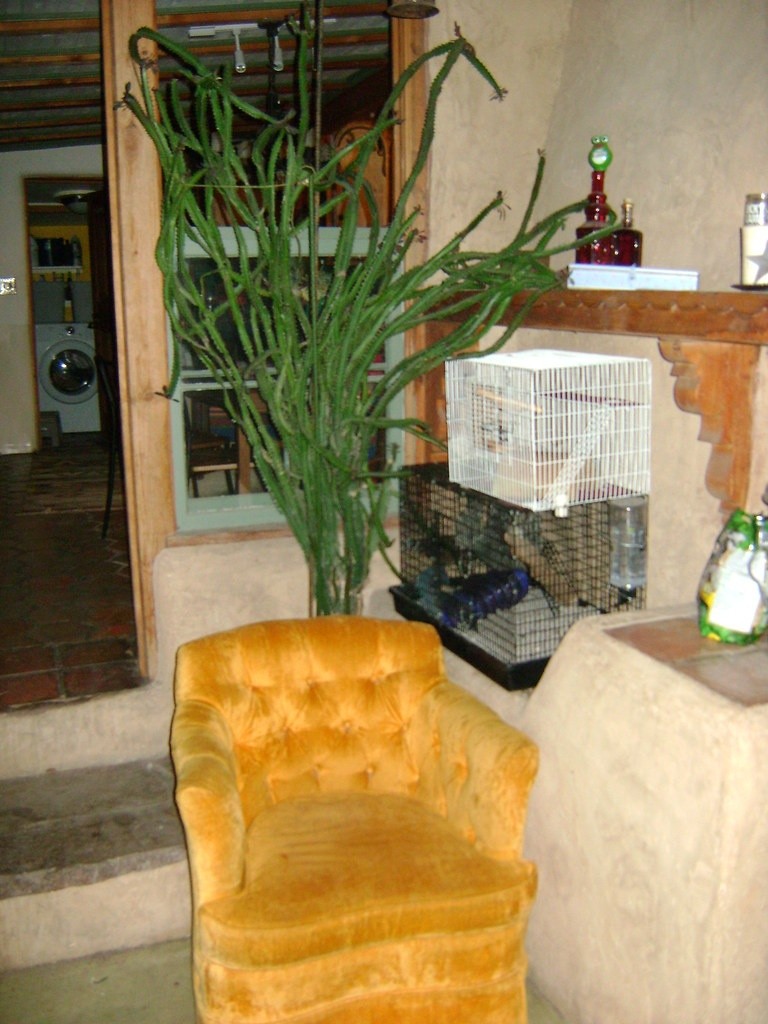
[169,614,544,1024]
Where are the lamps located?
[52,188,98,215]
[189,17,337,74]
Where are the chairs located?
[95,353,236,539]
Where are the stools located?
[39,411,62,450]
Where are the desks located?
[518,603,768,1024]
[180,364,391,494]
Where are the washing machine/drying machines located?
[35,321,99,433]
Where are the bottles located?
[576,170,615,266]
[613,197,643,267]
